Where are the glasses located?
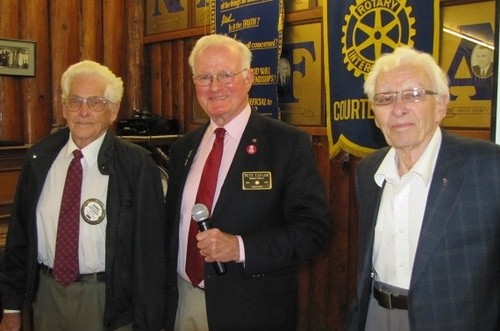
[193,69,247,86]
[63,94,110,112]
[374,88,437,106]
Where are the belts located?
[373,286,408,310]
[39,263,105,283]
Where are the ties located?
[53,150,84,287]
[185,128,226,289]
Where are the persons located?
[0,45,30,69]
[2,59,173,331]
[159,34,336,331]
[345,42,500,331]
[471,44,494,78]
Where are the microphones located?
[190,204,228,275]
